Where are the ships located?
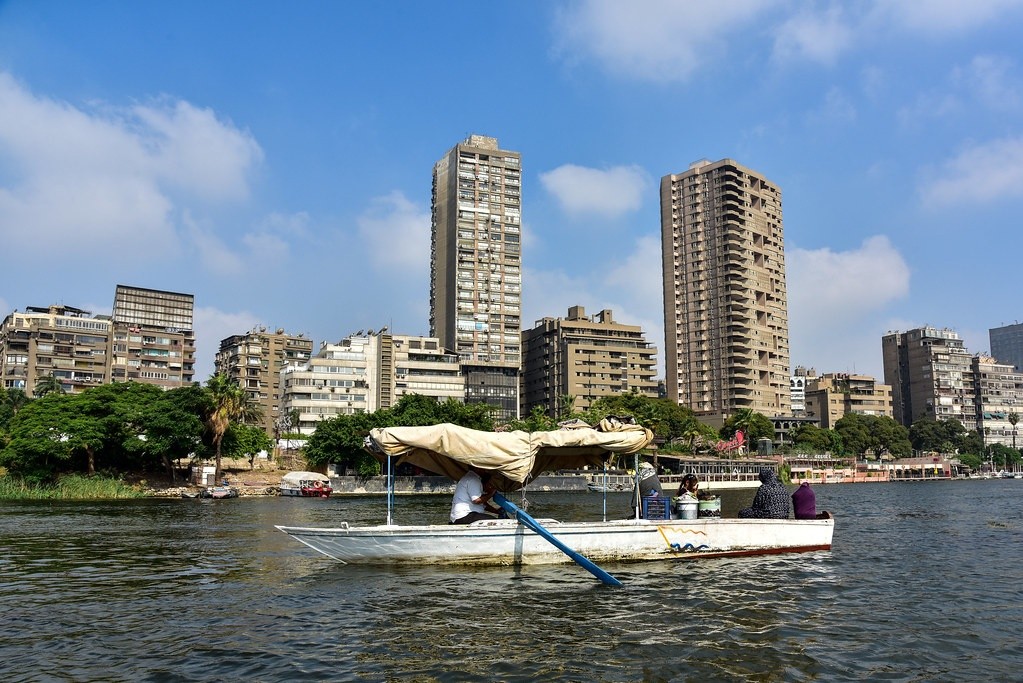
[586,451,778,493]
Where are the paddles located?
[489,488,622,586]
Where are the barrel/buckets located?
[677,500,699,520]
[699,498,722,519]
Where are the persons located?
[737,468,790,519]
[449,462,503,526]
[676,474,706,513]
[791,482,830,520]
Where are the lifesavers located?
[314,480,322,489]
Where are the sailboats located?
[952,448,1023,480]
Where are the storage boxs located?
[642,497,669,520]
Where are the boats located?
[276,420,837,567]
[180,486,243,498]
[279,471,332,498]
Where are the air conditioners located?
[319,344,406,419]
[458,166,497,361]
[49,338,149,382]
